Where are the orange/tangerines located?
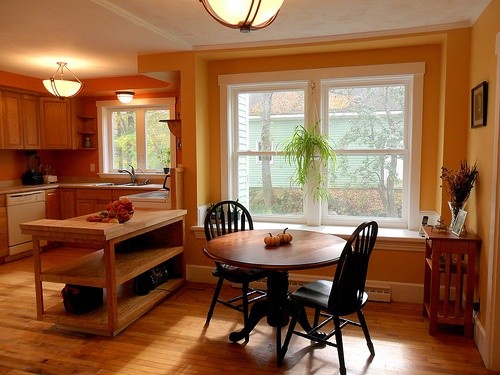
[109,218,119,223]
[121,198,128,204]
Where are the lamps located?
[41,62,86,101]
[199,0,284,35]
[115,90,135,103]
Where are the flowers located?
[438,158,479,225]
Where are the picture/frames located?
[471,81,489,128]
[451,209,468,236]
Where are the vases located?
[447,201,467,233]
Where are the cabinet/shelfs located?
[0,194,8,262]
[0,90,40,150]
[77,199,112,217]
[39,92,97,151]
[46,189,61,249]
[421,226,483,339]
[59,189,76,246]
[19,207,188,339]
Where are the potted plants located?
[273,119,337,226]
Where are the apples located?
[86,197,135,223]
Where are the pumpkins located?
[264,228,293,245]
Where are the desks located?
[203,229,355,369]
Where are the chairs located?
[204,199,268,342]
[281,221,379,375]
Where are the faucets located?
[118,164,136,183]
[136,168,148,184]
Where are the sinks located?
[93,183,125,186]
[125,184,149,186]
